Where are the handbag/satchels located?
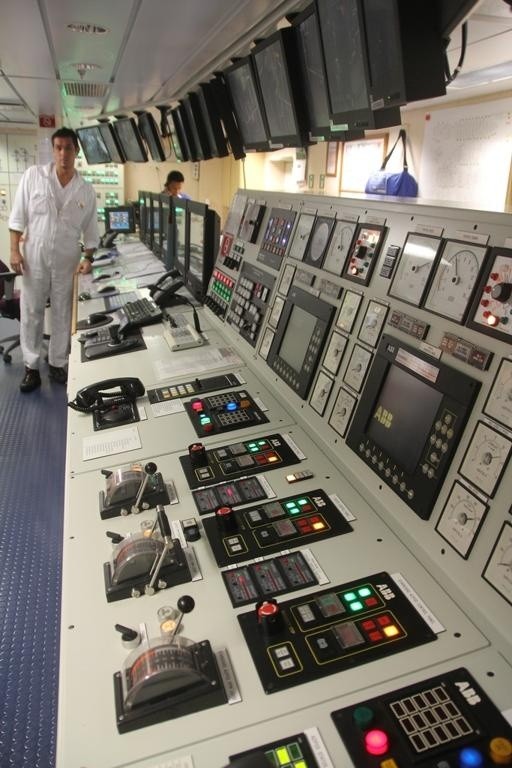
[364,129,418,197]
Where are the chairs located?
[0,258,51,365]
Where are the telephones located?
[150,269,188,307]
[77,377,145,407]
[98,229,118,249]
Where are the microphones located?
[147,285,204,332]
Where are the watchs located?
[85,255,95,263]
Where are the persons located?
[160,169,192,199]
[9,128,98,393]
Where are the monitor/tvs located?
[344,333,483,523]
[75,1,448,166]
[138,190,221,308]
[103,206,136,234]
[266,285,338,402]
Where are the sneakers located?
[48,364,68,385]
[19,364,42,392]
